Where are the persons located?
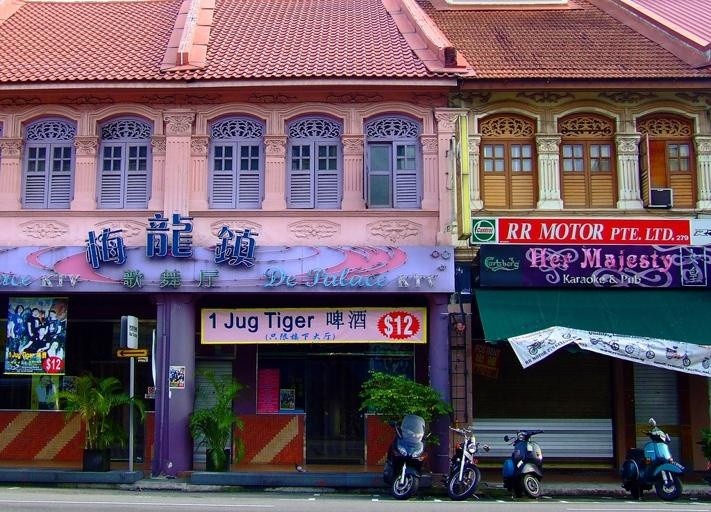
[7,303,67,360]
[36,375,57,410]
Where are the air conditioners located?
[647,186,674,209]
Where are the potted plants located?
[46,375,147,471]
[185,367,246,473]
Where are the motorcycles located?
[498,427,546,499]
[441,424,491,501]
[383,413,433,500]
[617,416,686,502]
